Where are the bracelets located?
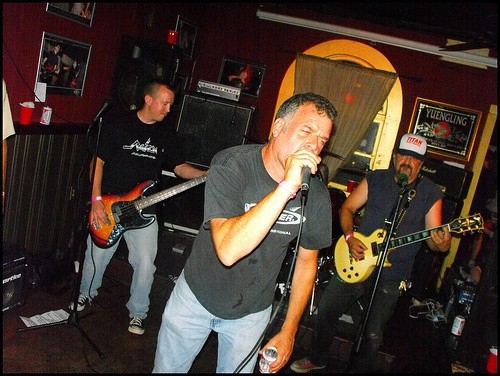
[91,196,103,202]
[345,233,354,242]
[280,182,297,199]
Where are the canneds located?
[259,346,277,373]
[40,106,52,125]
[451,315,465,336]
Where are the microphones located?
[397,172,408,186]
[92,97,114,124]
[301,167,311,198]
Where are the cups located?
[18,102,35,125]
[168,29,178,44]
[347,180,361,193]
[485,348,498,374]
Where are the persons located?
[289,133,452,376]
[2,83,17,195]
[68,76,207,334]
[152,93,338,373]
[40,42,61,86]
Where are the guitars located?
[85,173,210,250]
[333,213,486,283]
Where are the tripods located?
[17,115,104,360]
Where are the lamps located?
[256,10,498,68]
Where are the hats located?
[396,133,428,160]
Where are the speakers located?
[173,90,256,171]
[414,198,461,287]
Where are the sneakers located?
[128,317,146,335]
[69,293,90,311]
[290,357,327,373]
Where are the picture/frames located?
[34,31,93,97]
[217,54,266,96]
[407,97,482,161]
[44,2,97,27]
